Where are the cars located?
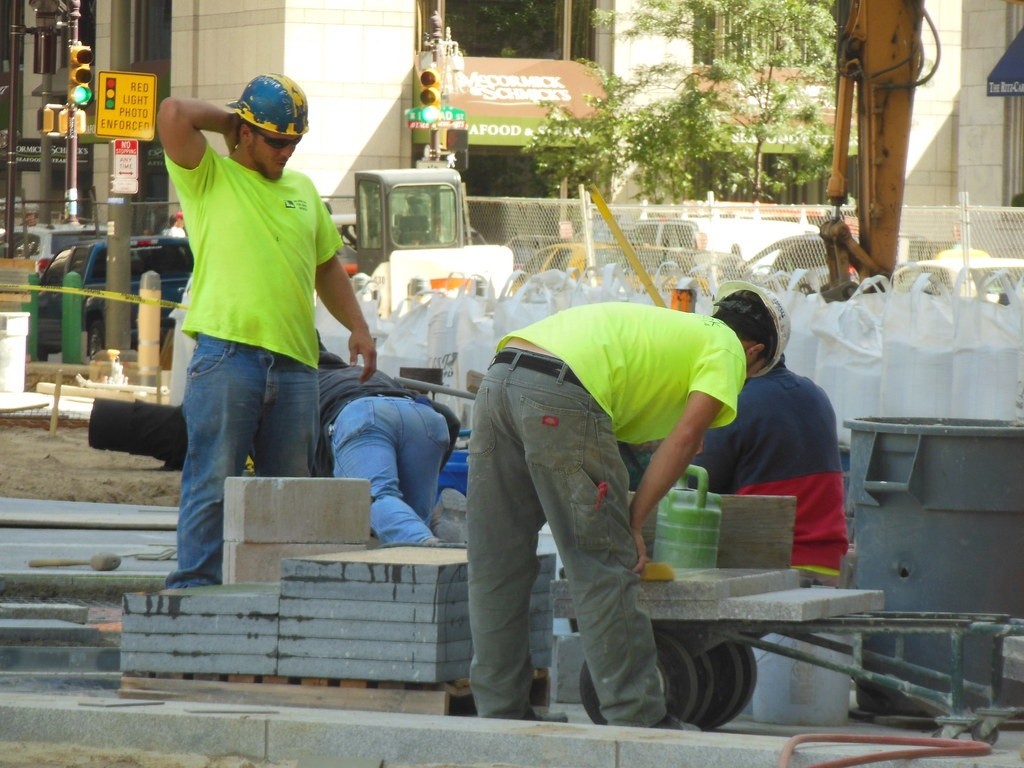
[328,214,1024,306]
[0,224,194,362]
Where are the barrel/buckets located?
[843,417,1024,711]
[749,631,853,723]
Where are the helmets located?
[226,74,309,136]
[713,281,791,378]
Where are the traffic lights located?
[68,44,93,106]
[446,129,469,172]
[35,107,54,134]
[420,68,440,124]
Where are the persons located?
[691,353,849,580]
[466,280,793,732]
[156,73,376,590]
[315,328,461,545]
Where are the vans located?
[637,218,818,274]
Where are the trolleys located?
[559,567,1024,747]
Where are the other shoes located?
[517,706,569,724]
[649,714,701,732]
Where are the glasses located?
[249,125,302,149]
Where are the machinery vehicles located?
[818,0,941,368]
[354,169,514,318]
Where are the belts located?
[486,352,588,392]
